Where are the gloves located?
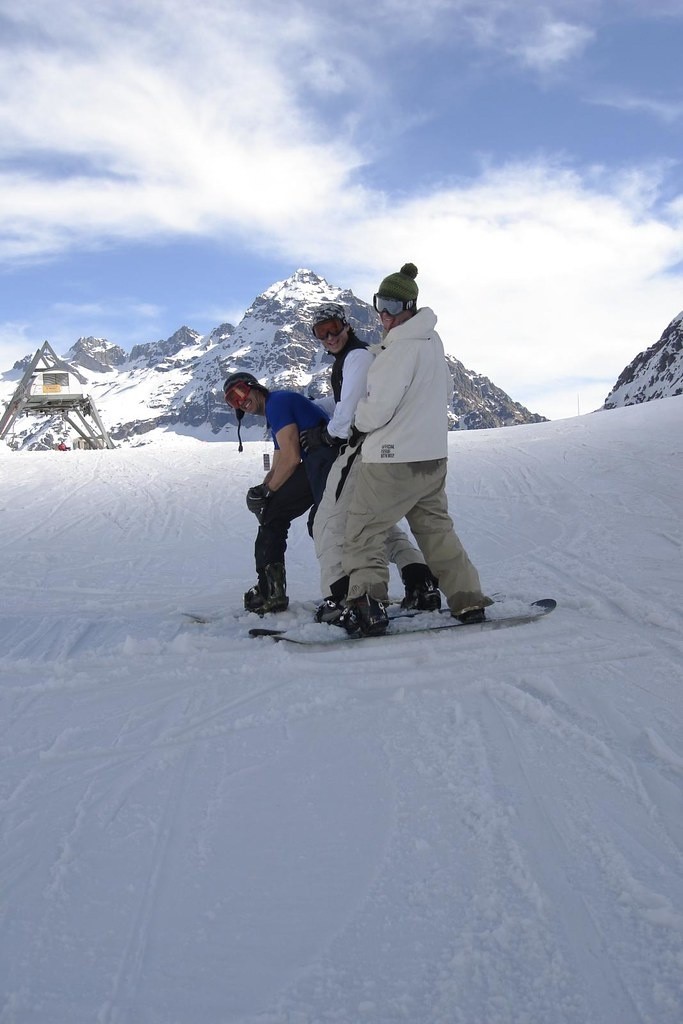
[299,422,338,454]
[245,484,273,515]
[349,424,364,448]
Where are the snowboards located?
[246,586,510,637]
[179,597,405,623]
[269,595,557,645]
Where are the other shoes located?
[451,610,485,624]
[335,596,389,635]
[400,581,441,609]
[243,584,287,613]
[315,592,347,621]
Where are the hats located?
[313,303,346,324]
[378,262,419,312]
[223,372,260,452]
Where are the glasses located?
[314,318,344,339]
[377,297,403,315]
[224,382,250,409]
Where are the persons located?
[222,371,352,615]
[340,261,494,639]
[306,302,441,625]
